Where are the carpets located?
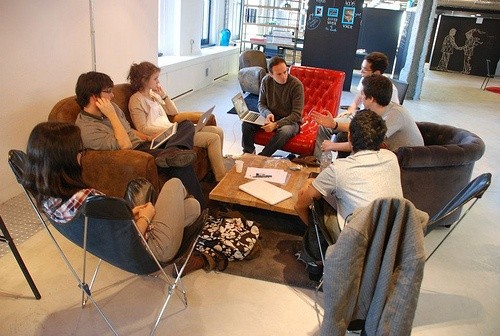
[176,151,323,292]
[226,92,259,114]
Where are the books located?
[239,166,293,205]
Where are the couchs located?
[397,120,486,229]
[237,49,269,95]
[47,82,217,197]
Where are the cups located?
[235,160,244,173]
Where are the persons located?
[23,122,208,279]
[311,74,425,158]
[242,56,304,157]
[75,71,207,210]
[127,61,226,185]
[313,52,401,159]
[294,108,403,268]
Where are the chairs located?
[481,58,500,91]
[7,146,210,336]
[311,168,493,297]
[253,65,346,155]
[392,77,409,106]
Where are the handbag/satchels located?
[194,206,261,271]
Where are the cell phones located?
[308,172,319,177]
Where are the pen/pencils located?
[253,175,272,178]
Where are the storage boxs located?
[273,37,292,45]
[272,30,291,37]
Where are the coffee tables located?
[209,151,319,217]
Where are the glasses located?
[100,87,114,95]
[361,65,372,71]
[76,147,88,157]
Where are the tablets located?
[150,122,177,151]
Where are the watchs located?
[274,122,280,129]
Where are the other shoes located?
[156,148,198,167]
[293,240,323,273]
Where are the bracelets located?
[135,215,150,225]
[162,95,169,101]
[332,122,338,130]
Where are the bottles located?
[320,150,332,172]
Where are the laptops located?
[231,92,266,126]
[195,105,216,132]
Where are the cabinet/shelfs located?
[237,0,303,65]
